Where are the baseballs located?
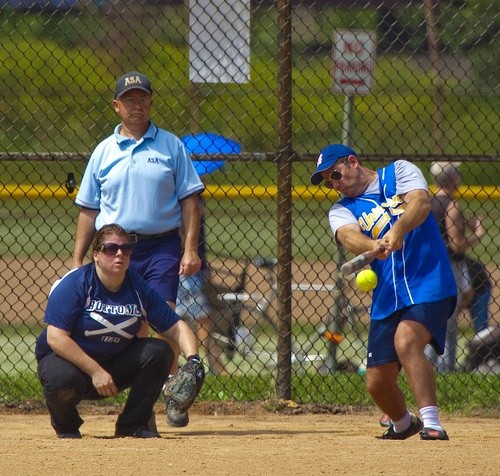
[356,269,377,291]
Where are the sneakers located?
[165,394,189,428]
[375,412,423,440]
[418,428,450,441]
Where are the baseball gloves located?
[162,356,205,412]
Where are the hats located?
[115,72,153,99]
[311,144,357,185]
[430,162,462,184]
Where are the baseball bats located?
[340,244,386,276]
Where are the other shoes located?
[114,429,163,438]
[58,430,82,439]
[471,326,495,348]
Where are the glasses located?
[95,243,136,257]
[324,159,346,190]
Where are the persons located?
[72,72,205,427]
[424,161,495,370]
[310,145,457,440]
[175,190,227,374]
[34,224,205,439]
